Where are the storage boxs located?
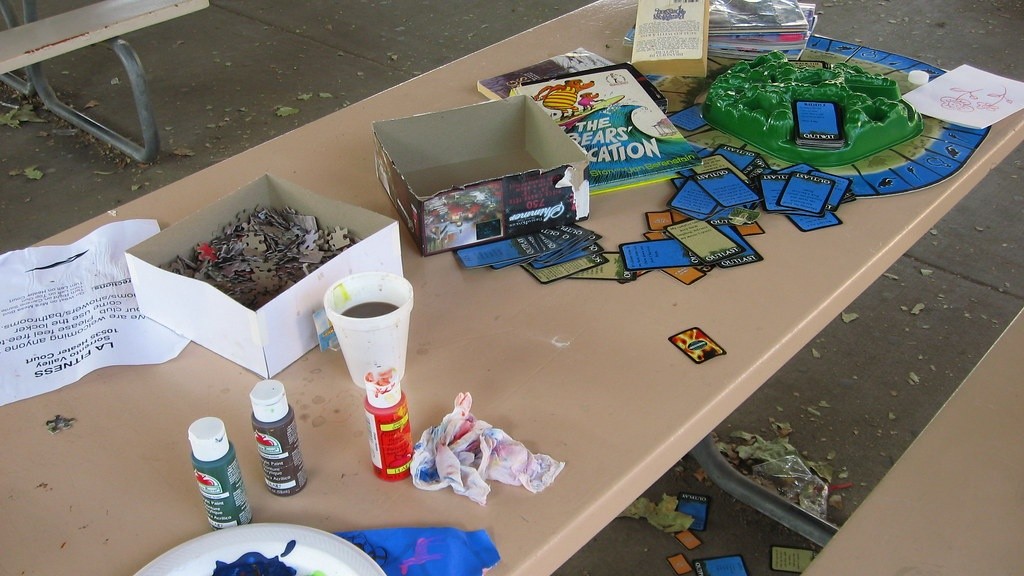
[371,94,591,257]
[124,172,403,379]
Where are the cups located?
[324,271,416,388]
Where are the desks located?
[0,0,1024,576]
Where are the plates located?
[132,522,386,576]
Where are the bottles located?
[249,378,307,497]
[363,364,414,481]
[187,417,254,531]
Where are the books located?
[477,46,704,196]
[622,0,819,78]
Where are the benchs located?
[796,306,1024,576]
[0,0,218,162]
